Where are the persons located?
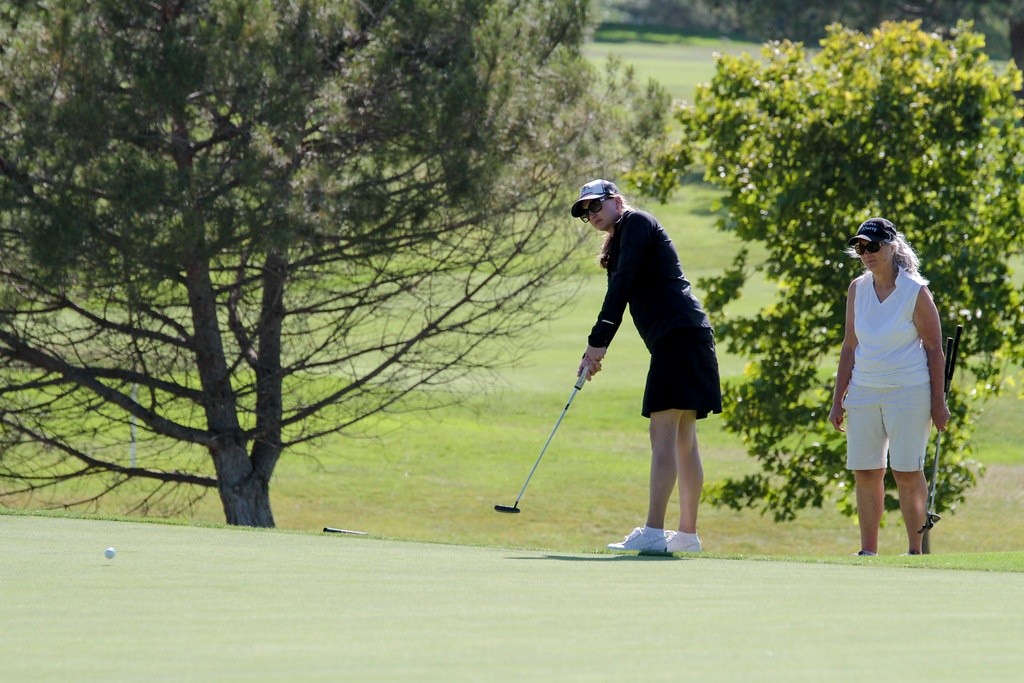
[829,218,951,556]
[571,179,724,554]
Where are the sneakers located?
[664,530,701,553]
[607,527,667,551]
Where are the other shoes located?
[858,545,877,556]
[907,549,922,554]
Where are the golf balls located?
[104,547,116,560]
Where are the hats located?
[571,179,619,218]
[847,218,896,245]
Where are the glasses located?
[855,242,885,255]
[579,197,613,223]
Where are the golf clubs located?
[915,323,963,536]
[494,365,589,515]
